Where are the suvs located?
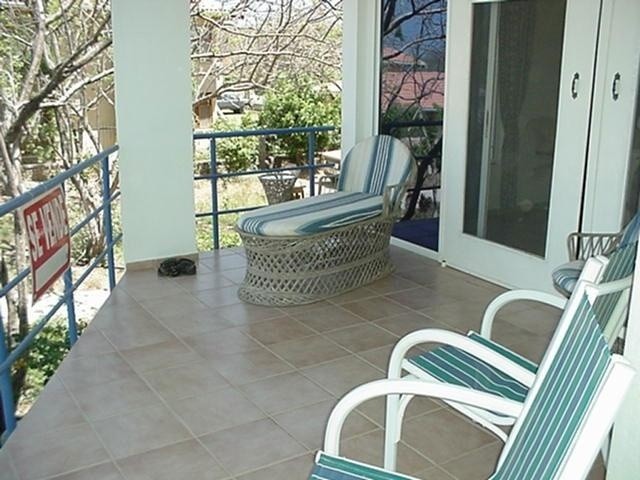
[217,80,269,114]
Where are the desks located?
[322,149,341,169]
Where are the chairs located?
[233,134,416,308]
[548,215,639,309]
[308,239,639,478]
[318,173,340,195]
